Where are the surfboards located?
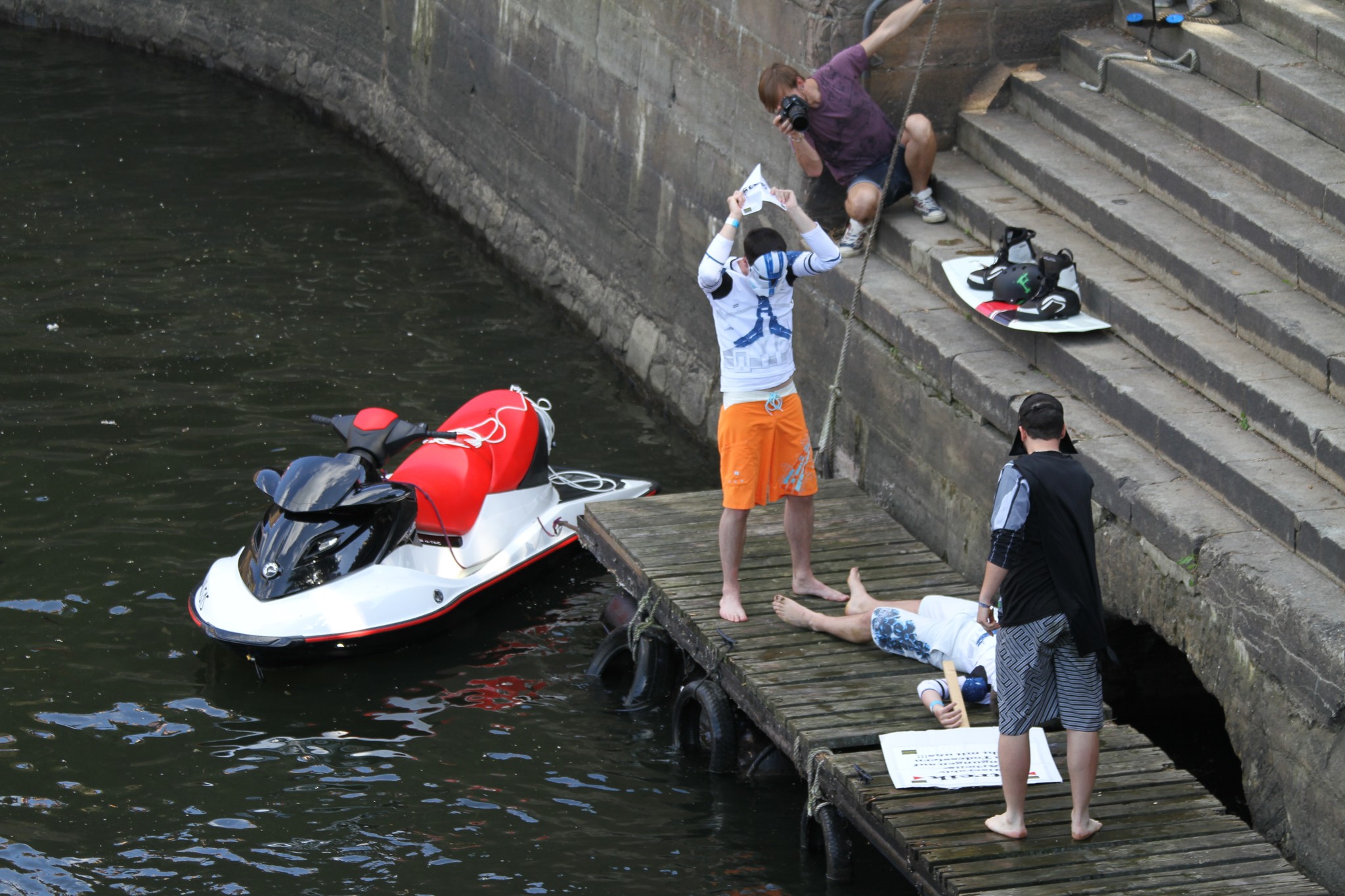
[941,256,1112,333]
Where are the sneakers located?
[909,186,947,223]
[1010,251,1082,320]
[967,227,1039,291]
[837,217,874,258]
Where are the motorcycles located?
[186,384,663,647]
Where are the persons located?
[697,187,850,623]
[771,566,998,730]
[757,0,947,259]
[976,393,1107,842]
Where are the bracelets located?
[724,217,740,229]
[789,132,805,142]
[929,700,944,714]
[978,601,990,608]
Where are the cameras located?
[777,94,811,133]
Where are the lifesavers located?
[582,620,685,705]
[669,678,737,778]
[798,794,848,880]
